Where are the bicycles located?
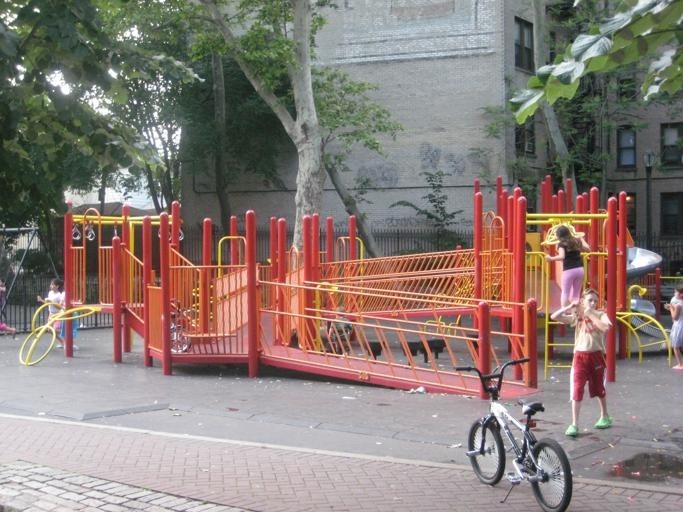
[171,301,192,353]
[454,358,572,512]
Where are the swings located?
[0,230,20,309]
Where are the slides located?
[587,243,672,339]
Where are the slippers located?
[564,425,578,436]
[672,364,683,369]
[594,416,612,428]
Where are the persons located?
[0,321,17,339]
[36,277,64,349]
[0,278,6,306]
[543,225,591,315]
[550,287,614,437]
[663,285,683,371]
[59,285,79,352]
